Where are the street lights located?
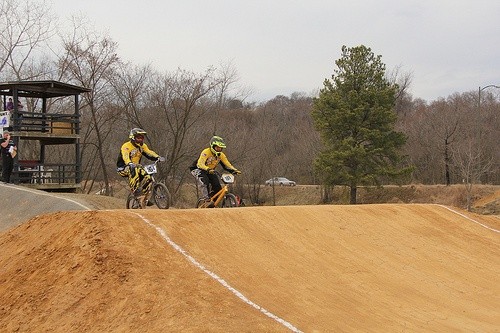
[475,83,500,183]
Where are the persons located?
[7,98,24,124]
[117,127,165,206]
[0,130,17,183]
[190,135,241,208]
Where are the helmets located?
[210,135,226,156]
[129,127,147,146]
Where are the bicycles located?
[126,157,171,209]
[195,170,244,208]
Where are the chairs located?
[24,166,53,184]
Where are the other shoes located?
[146,199,154,206]
[134,189,146,198]
[205,199,214,207]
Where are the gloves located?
[159,156,165,162]
[234,170,241,174]
[128,161,136,167]
[209,169,215,174]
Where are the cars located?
[265,177,296,187]
